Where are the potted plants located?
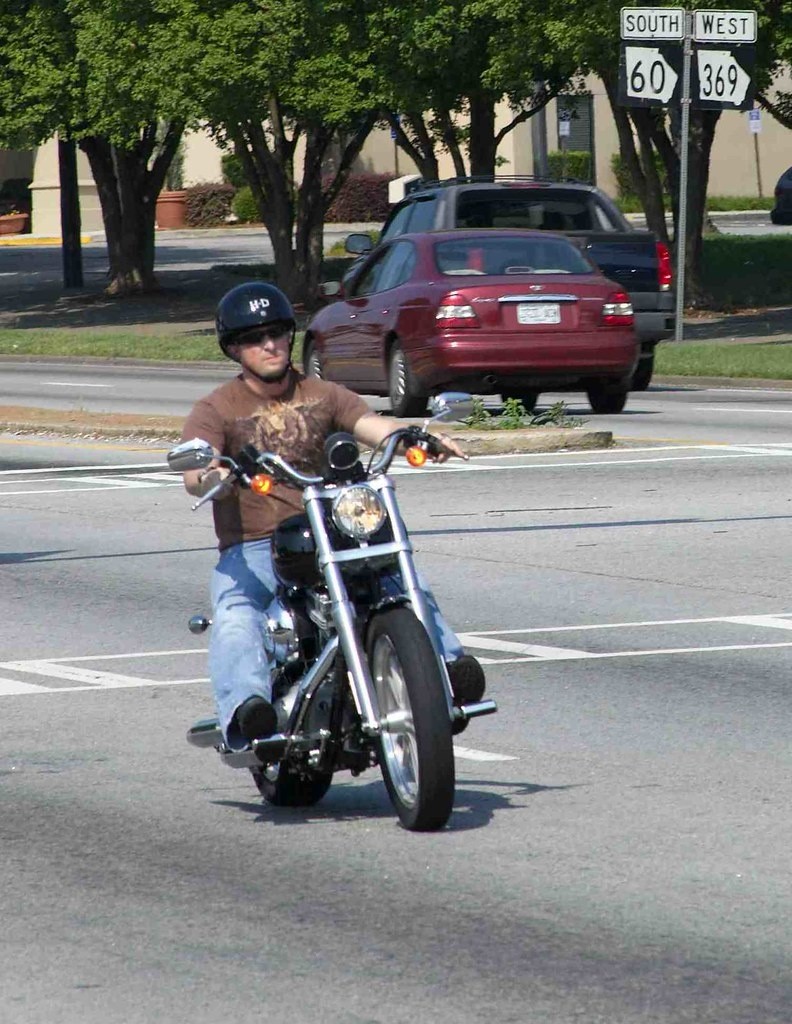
[154,124,189,228]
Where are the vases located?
[0,213,29,234]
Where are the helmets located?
[215,282,296,349]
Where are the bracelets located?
[198,465,215,485]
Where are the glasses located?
[237,319,294,346]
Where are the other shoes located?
[449,655,484,736]
[237,696,277,740]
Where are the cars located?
[301,229,642,417]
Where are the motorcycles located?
[166,392,499,831]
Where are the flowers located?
[0,208,24,217]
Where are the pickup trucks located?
[343,175,678,390]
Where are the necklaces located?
[180,284,486,752]
